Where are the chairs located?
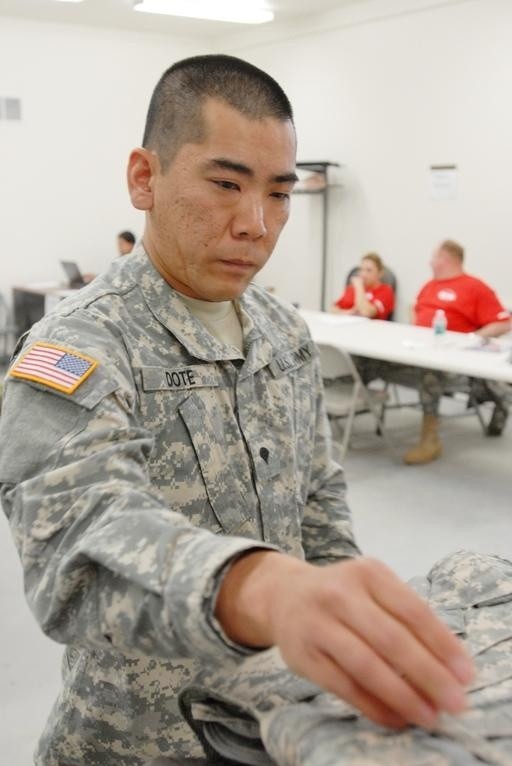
[346,263,396,320]
[374,308,512,436]
[319,341,399,464]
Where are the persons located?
[310,250,395,389]
[0,51,476,765]
[117,232,135,255]
[399,238,512,466]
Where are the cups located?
[437,333,456,357]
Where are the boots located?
[404,413,441,465]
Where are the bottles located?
[432,310,448,356]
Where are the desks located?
[288,302,512,384]
[13,275,89,341]
